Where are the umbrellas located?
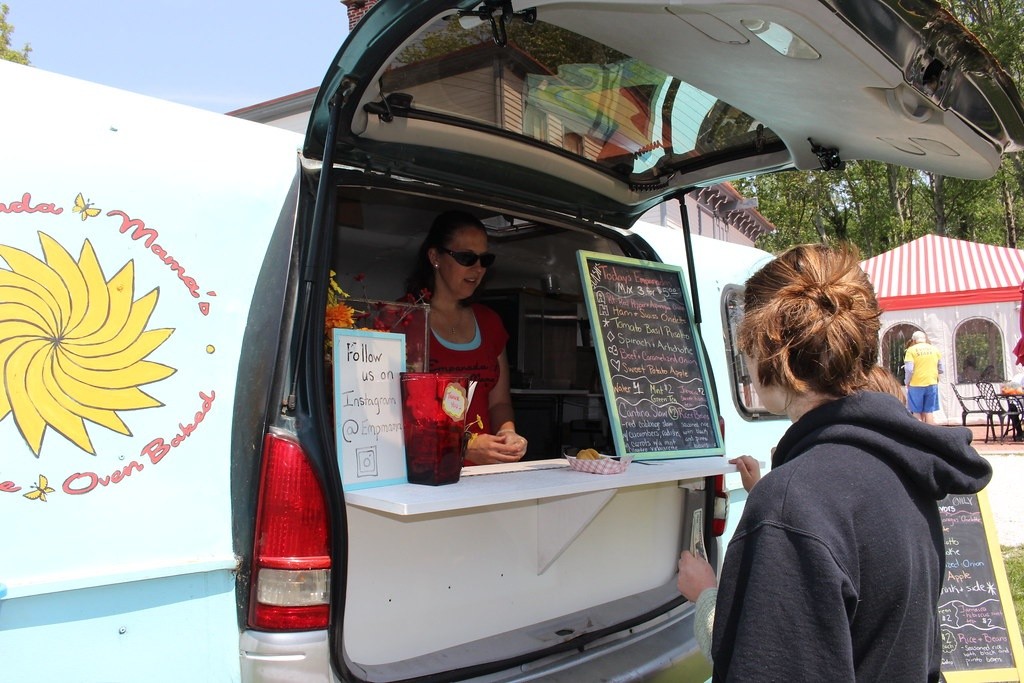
[1012,281,1024,367]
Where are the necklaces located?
[447,317,461,335]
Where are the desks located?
[994,393,1024,438]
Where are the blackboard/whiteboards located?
[576,248,727,463]
[332,326,409,494]
[939,493,1024,683]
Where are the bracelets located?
[468,433,478,446]
[496,429,515,436]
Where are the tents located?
[858,234,1024,424]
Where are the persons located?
[958,354,979,384]
[904,330,944,425]
[677,243,993,683]
[373,210,528,467]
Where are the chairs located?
[949,383,1006,441]
[976,381,1022,443]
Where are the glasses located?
[442,248,496,268]
